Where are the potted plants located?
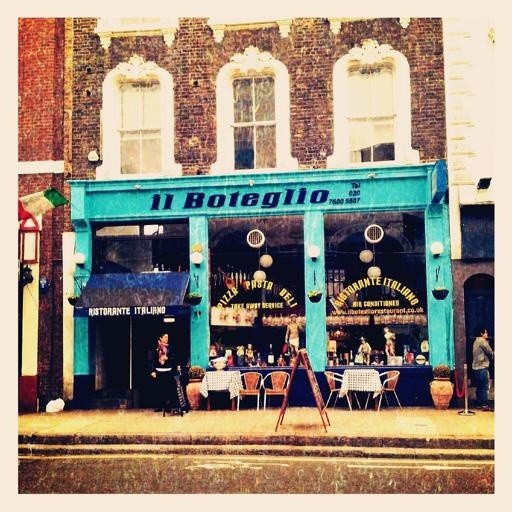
[185,365,205,409]
[428,365,455,410]
[431,287,449,300]
[185,292,202,306]
[65,292,79,306]
[307,290,322,303]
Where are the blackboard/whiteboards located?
[300,349,326,415]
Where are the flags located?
[18,187,69,222]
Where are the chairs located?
[364,370,403,412]
[323,372,361,412]
[263,371,290,410]
[236,372,263,411]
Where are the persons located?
[244,344,255,368]
[471,327,494,411]
[356,335,371,366]
[285,313,304,366]
[369,356,384,366]
[276,354,286,368]
[383,326,396,357]
[151,331,182,413]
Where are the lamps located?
[72,253,85,268]
[478,178,491,189]
[253,221,274,282]
[189,253,203,267]
[430,241,443,258]
[307,246,320,263]
[359,217,381,280]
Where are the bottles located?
[267,344,275,366]
[278,354,285,367]
[349,350,354,366]
